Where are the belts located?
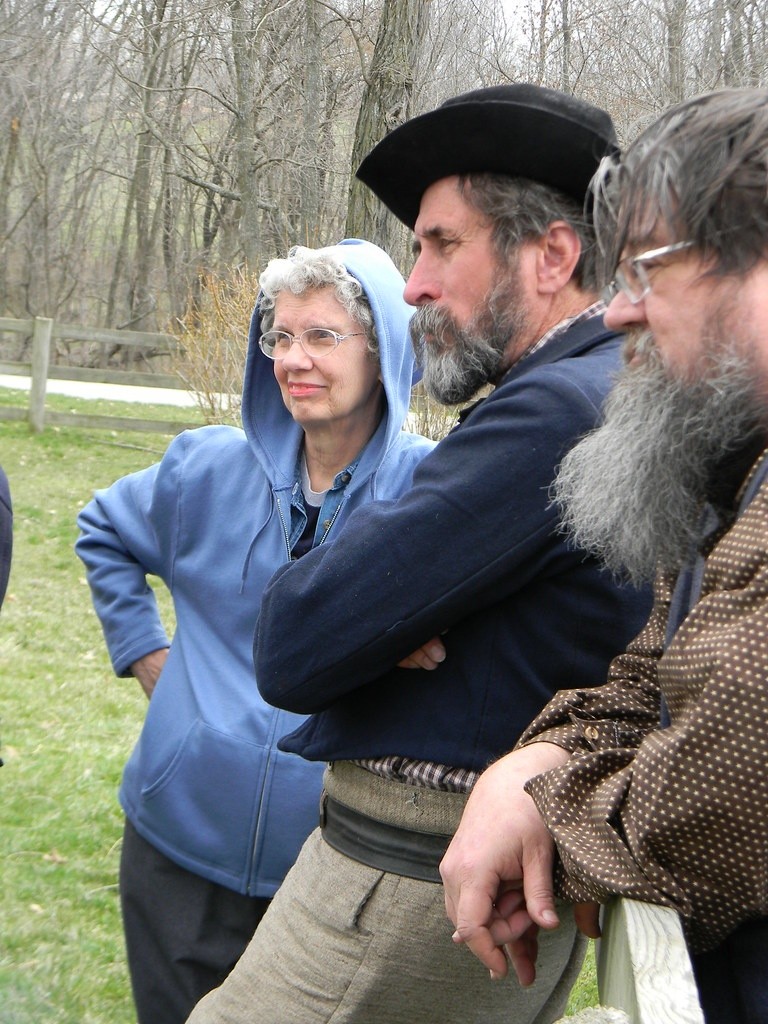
[322,793,454,884]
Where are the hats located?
[355,83,623,233]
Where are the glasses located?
[258,328,367,360]
[610,223,756,305]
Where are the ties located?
[659,457,768,730]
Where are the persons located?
[180,78,720,1024]
[75,242,441,1024]
[438,84,768,1024]
